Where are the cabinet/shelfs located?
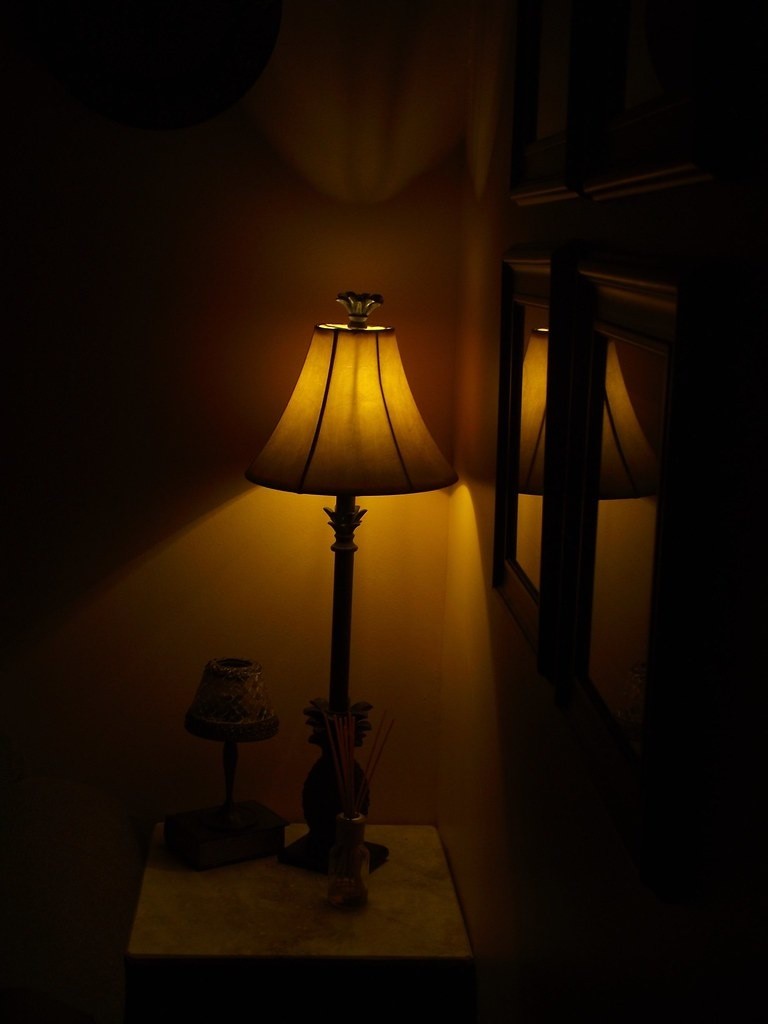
[123,820,479,1023]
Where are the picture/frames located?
[490,0,735,904]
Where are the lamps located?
[183,658,280,832]
[517,325,659,499]
[243,292,461,879]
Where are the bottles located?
[330,812,370,908]
